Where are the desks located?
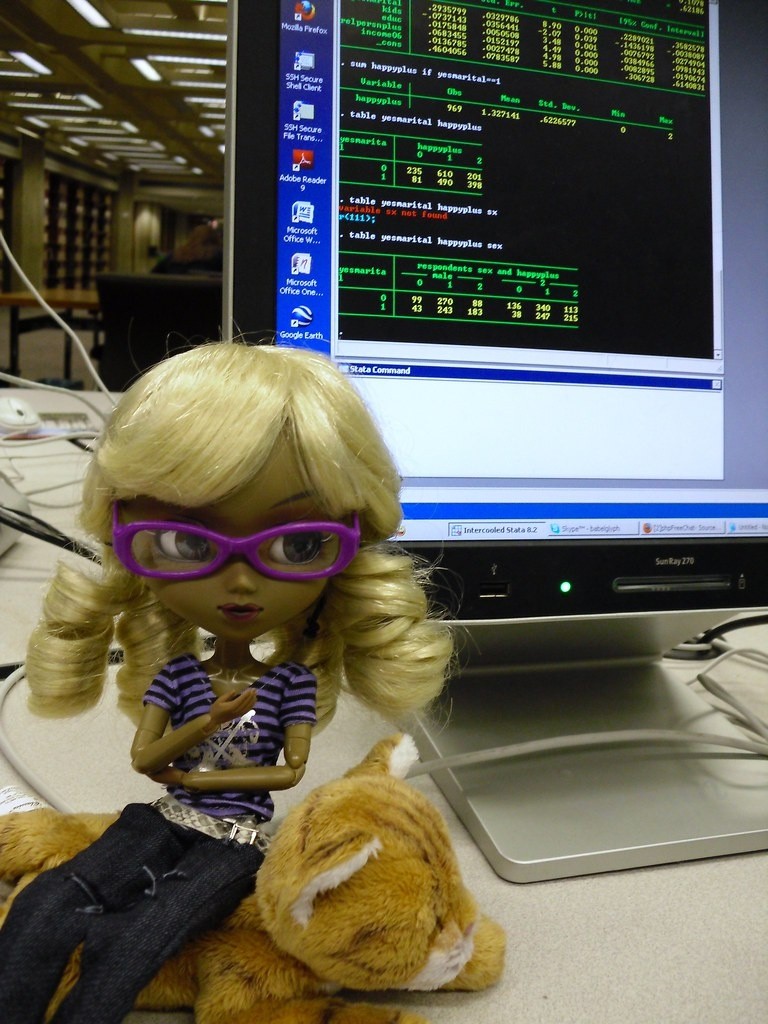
[0,392,768,1022]
[0,289,101,391]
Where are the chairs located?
[88,274,225,392]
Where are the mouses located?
[0,396,42,434]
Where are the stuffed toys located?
[0,731,508,1024]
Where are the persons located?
[2,339,456,1022]
[150,218,225,277]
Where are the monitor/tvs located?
[220,0,768,884]
